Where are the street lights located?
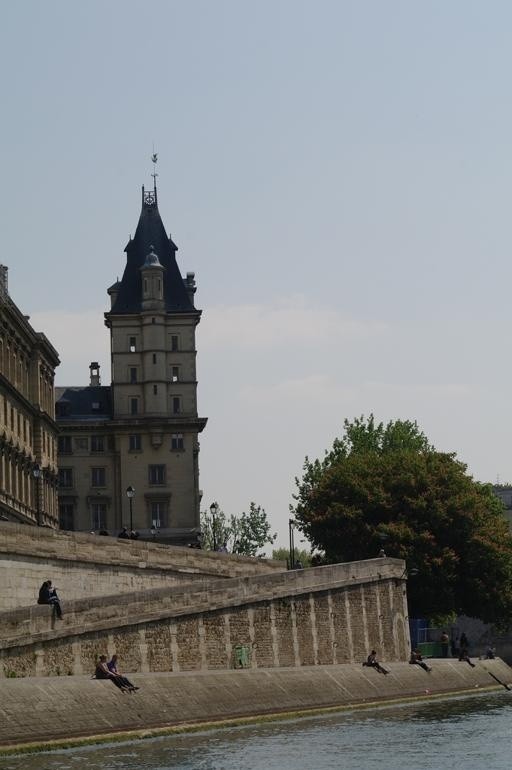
[126,485,135,532]
[150,524,156,542]
[209,502,217,551]
[290,519,296,569]
[32,464,41,527]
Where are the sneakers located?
[121,686,140,691]
[56,616,64,620]
[384,671,391,675]
[426,666,432,672]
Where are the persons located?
[378,548,386,558]
[295,559,304,569]
[47,579,59,600]
[410,647,431,673]
[217,542,229,553]
[439,627,497,668]
[362,648,391,677]
[88,526,143,540]
[95,653,128,692]
[36,583,64,622]
[108,654,140,690]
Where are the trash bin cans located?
[234,645,250,669]
